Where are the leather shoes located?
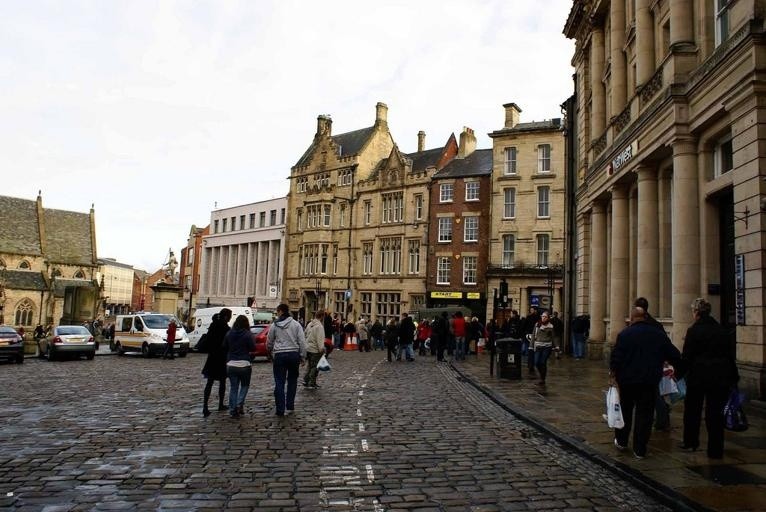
[386,350,476,364]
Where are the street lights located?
[345,163,359,323]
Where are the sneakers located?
[632,448,648,459]
[203,403,244,418]
[308,380,320,389]
[614,437,627,450]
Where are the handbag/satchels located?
[664,378,687,405]
[720,382,748,432]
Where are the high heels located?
[677,440,699,451]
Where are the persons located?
[297,308,562,387]
[265,303,308,416]
[18,323,25,340]
[610,307,666,458]
[675,297,739,459]
[163,317,177,360]
[33,323,44,338]
[201,308,233,417]
[569,314,590,359]
[223,314,257,416]
[602,297,681,432]
[80,313,115,350]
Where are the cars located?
[0,326,24,363]
[250,324,334,363]
[39,325,95,361]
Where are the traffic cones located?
[343,332,359,351]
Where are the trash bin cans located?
[495,338,522,380]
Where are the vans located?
[183,307,254,350]
[114,314,190,358]
